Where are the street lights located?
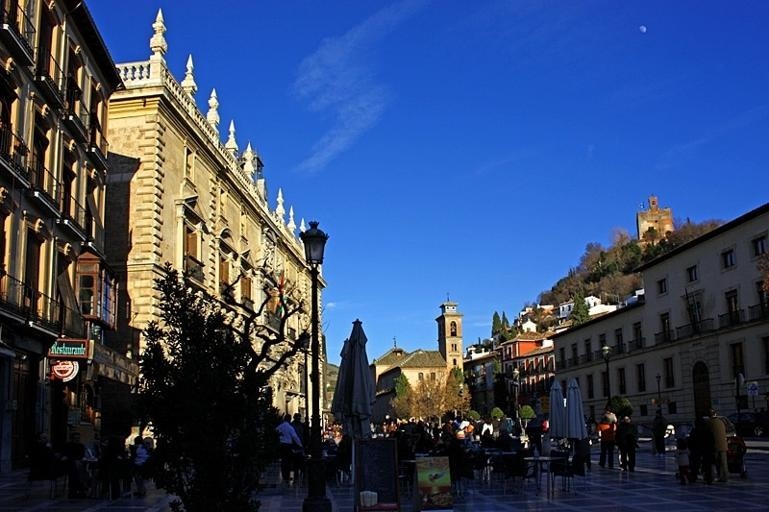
[512,369,520,420]
[655,374,661,405]
[601,345,613,409]
[300,221,329,464]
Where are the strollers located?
[728,434,748,479]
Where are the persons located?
[63,412,759,498]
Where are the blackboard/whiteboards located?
[354,438,400,504]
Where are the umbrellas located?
[562,377,588,441]
[548,379,566,443]
[329,318,374,486]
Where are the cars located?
[525,413,592,440]
[727,412,769,437]
